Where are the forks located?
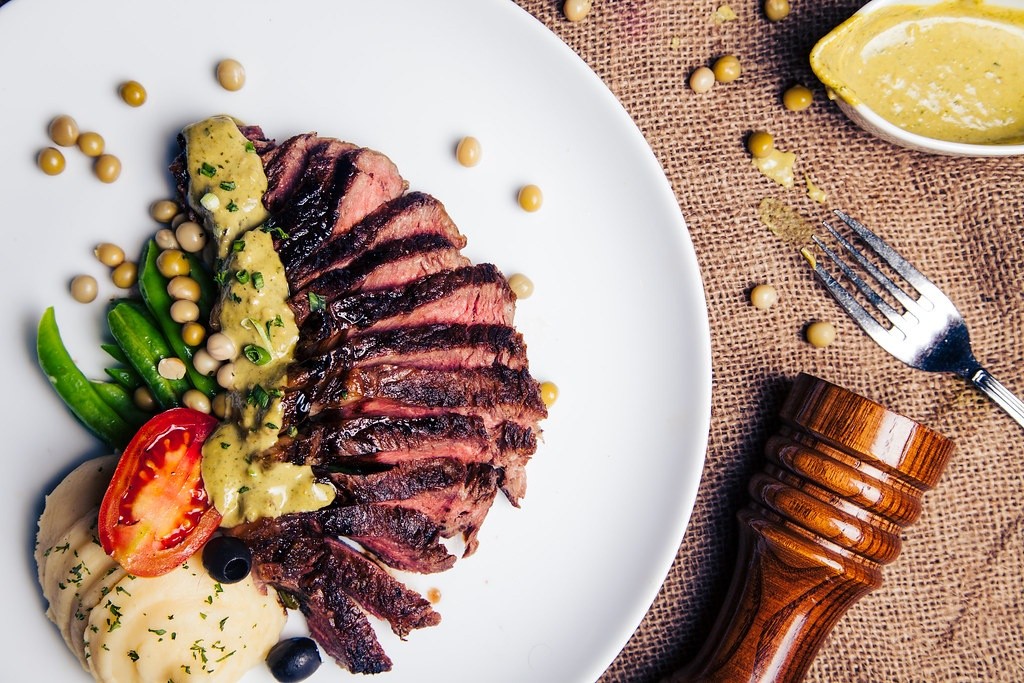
[800,208,1024,436]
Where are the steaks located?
[236,127,548,676]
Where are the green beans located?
[807,322,835,346]
[37,60,246,455]
[690,68,715,92]
[508,274,532,299]
[519,185,541,212]
[563,0,591,21]
[538,382,557,405]
[714,56,741,82]
[457,136,480,167]
[765,0,788,21]
[751,285,776,307]
[783,87,812,110]
[749,132,773,158]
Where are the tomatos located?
[97,409,221,576]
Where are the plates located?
[0,0,717,683]
[808,0,1024,157]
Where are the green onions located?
[197,143,326,410]
